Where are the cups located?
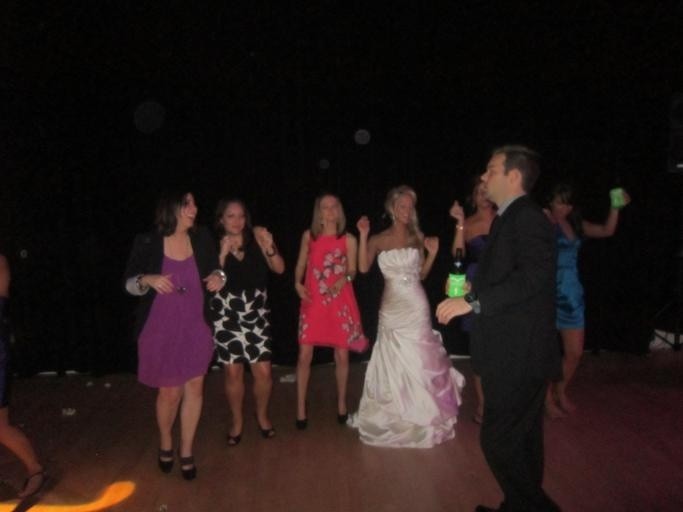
[609,188,624,208]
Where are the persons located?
[0,251,63,512]
[435,146,632,512]
[294,193,369,428]
[124,187,227,481]
[211,195,286,447]
[345,187,466,449]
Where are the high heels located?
[226,418,244,446]
[255,411,276,438]
[296,401,308,431]
[336,400,348,423]
[176,447,196,480]
[158,448,174,473]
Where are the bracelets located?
[265,246,276,257]
[347,275,352,282]
[135,276,147,291]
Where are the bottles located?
[448,248,466,298]
